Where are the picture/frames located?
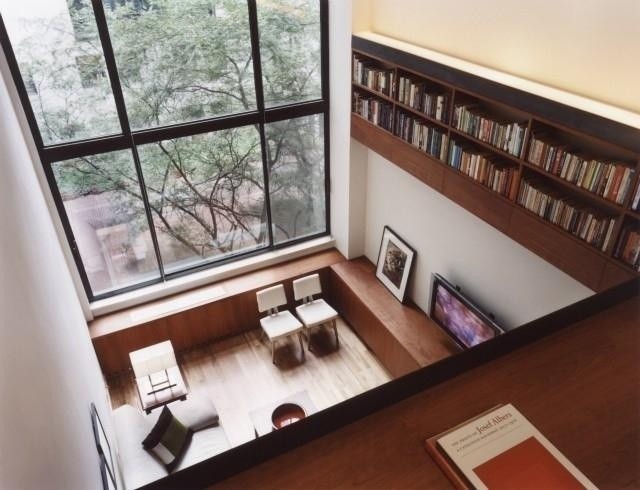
[375,225,416,304]
[91,402,117,490]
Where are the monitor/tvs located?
[426,272,506,351]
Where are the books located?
[422,400,599,490]
[447,105,529,199]
[352,59,397,133]
[394,77,450,162]
[518,138,639,265]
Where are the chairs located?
[111,394,232,490]
[255,272,339,364]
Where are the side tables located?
[128,339,189,414]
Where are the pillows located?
[141,403,195,474]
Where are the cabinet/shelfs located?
[351,37,640,293]
